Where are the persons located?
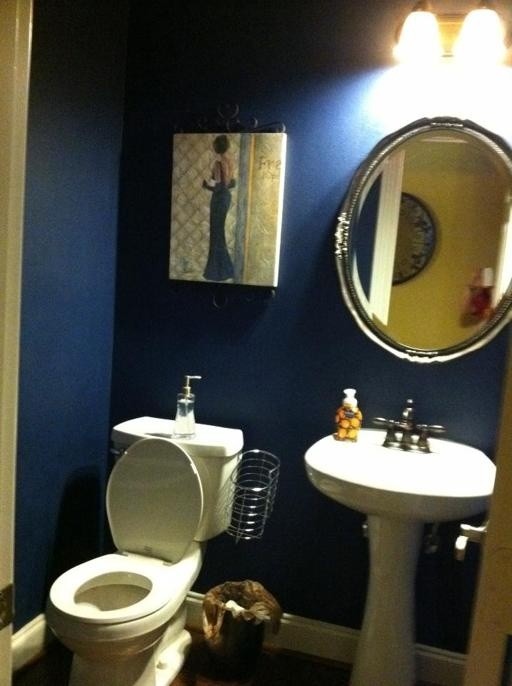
[200,135,236,281]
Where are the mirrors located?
[325,112,512,370]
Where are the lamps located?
[386,0,512,76]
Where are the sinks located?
[304,428,496,524]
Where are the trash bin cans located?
[203,579,282,680]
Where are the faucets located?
[396,399,414,448]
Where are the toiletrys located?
[175,375,202,433]
[335,388,362,442]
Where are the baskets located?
[225,449,280,541]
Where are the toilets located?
[44,414,245,685]
